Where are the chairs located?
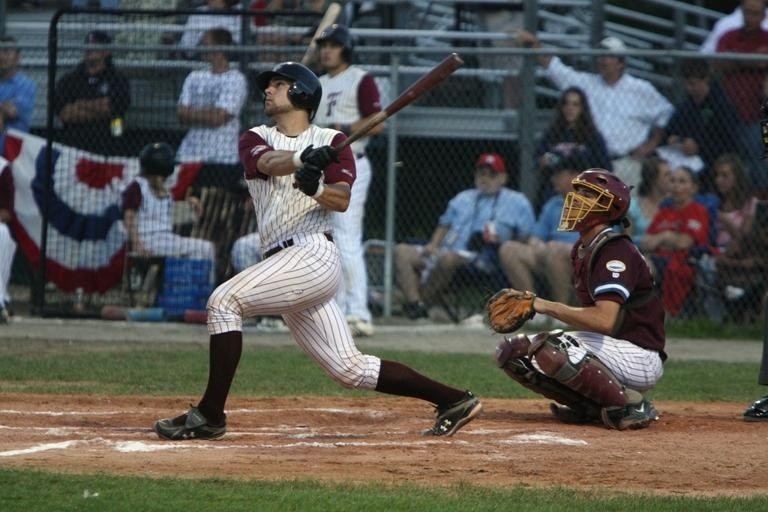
[394,237,500,326]
[120,198,204,310]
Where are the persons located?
[742,312,768,422]
[120,140,216,313]
[163,0,357,69]
[152,62,484,440]
[0,33,36,152]
[231,234,291,336]
[313,25,386,338]
[394,150,536,321]
[486,168,668,429]
[166,30,249,201]
[0,153,20,323]
[53,30,129,154]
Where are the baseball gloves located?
[487,288,536,333]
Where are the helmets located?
[255,61,323,122]
[314,22,355,62]
[556,167,636,233]
[139,141,181,176]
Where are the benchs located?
[0,23,354,134]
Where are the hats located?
[475,152,506,174]
[595,36,626,52]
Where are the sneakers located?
[421,388,482,438]
[549,398,660,432]
[742,394,768,422]
[343,297,568,338]
[153,402,227,441]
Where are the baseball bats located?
[334,52,463,150]
[300,3,341,66]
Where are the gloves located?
[293,162,324,200]
[298,143,340,171]
